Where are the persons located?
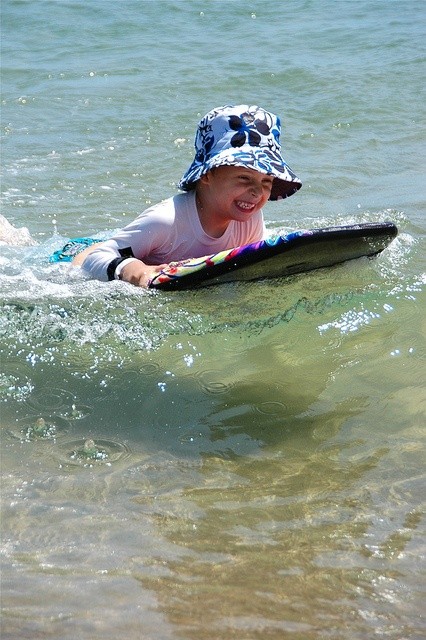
[49,104,302,290]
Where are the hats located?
[176,104,303,201]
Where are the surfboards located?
[147,222,399,290]
